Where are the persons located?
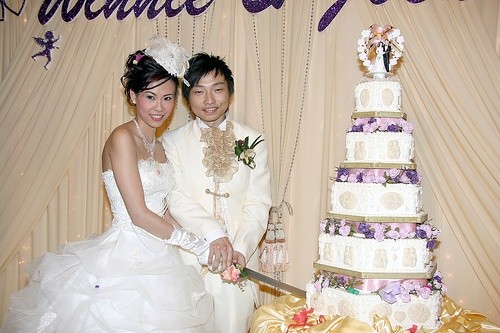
[8,34,223,333]
[383,39,391,72]
[157,53,272,333]
[373,41,387,78]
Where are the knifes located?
[229,262,306,299]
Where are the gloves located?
[164,225,221,273]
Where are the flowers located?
[221,262,247,292]
[235,135,264,169]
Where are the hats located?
[145,34,191,87]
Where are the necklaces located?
[133,118,156,158]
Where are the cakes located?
[305,80,447,331]
